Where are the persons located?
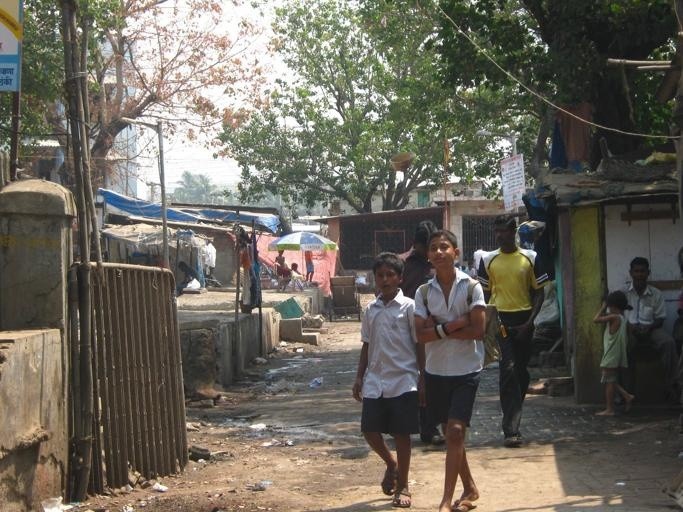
[607,255,680,405]
[481,213,548,447]
[394,220,447,445]
[414,229,488,512]
[175,261,198,298]
[593,289,634,418]
[352,251,426,508]
[274,249,317,290]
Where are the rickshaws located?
[326,273,361,324]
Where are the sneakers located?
[422,432,445,446]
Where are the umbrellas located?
[267,231,339,282]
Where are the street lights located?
[119,115,171,270]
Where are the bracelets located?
[434,323,449,340]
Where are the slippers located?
[504,429,525,445]
[381,462,398,496]
[394,488,411,508]
[451,496,480,512]
